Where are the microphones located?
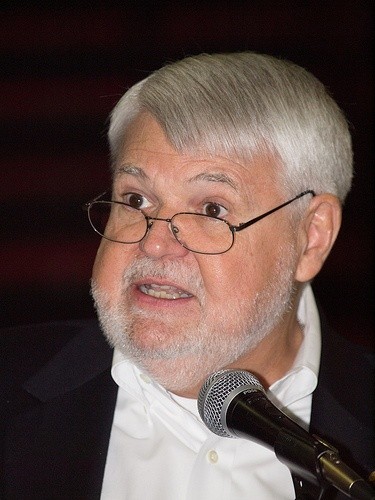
[198,368,369,499]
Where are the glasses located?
[84,190,318,255]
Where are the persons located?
[0,51,375,499]
